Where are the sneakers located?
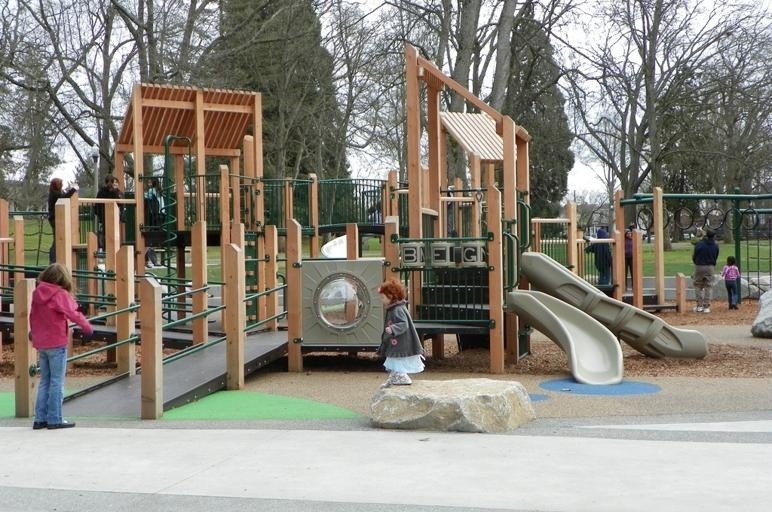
[393,376,413,385]
[33,421,46,429]
[47,420,75,429]
[703,308,711,313]
[693,306,703,312]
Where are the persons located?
[584,230,612,286]
[368,201,384,244]
[623,230,634,291]
[721,257,742,311]
[95,175,168,269]
[30,263,93,428]
[48,178,81,266]
[691,230,721,313]
[378,281,428,389]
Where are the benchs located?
[146,248,191,266]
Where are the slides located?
[320,234,347,259]
[507,251,706,386]
